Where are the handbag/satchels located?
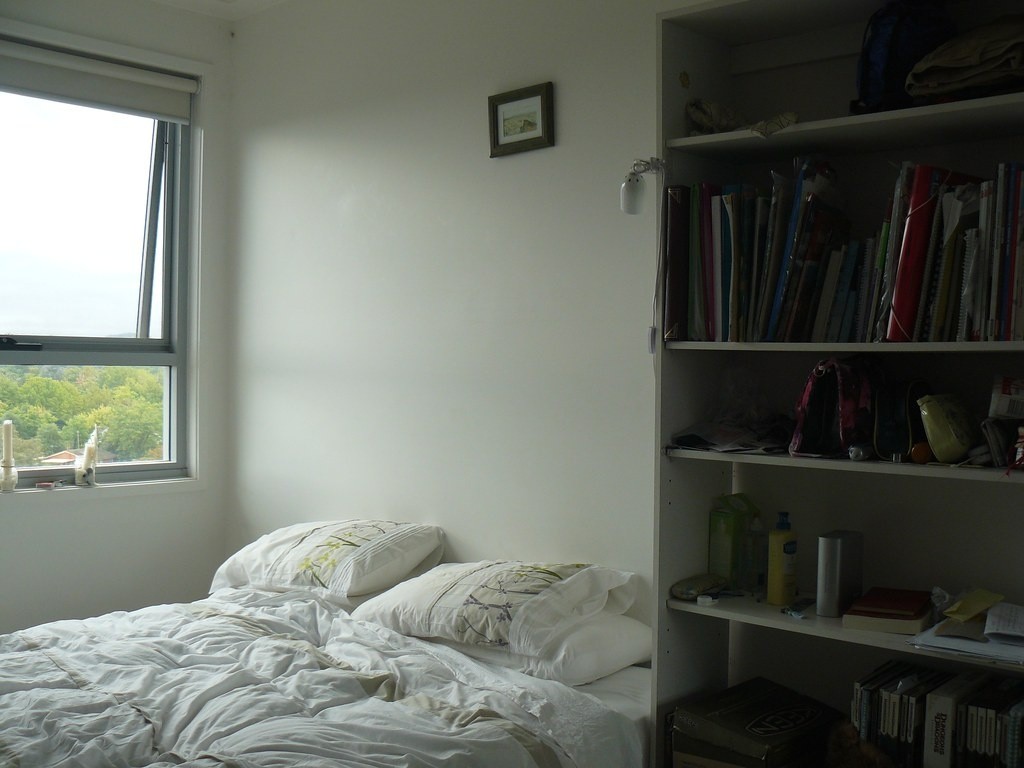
[787,358,878,457]
[899,379,987,466]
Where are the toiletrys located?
[767,509,799,608]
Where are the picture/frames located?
[488,82,555,159]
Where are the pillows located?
[209,521,447,607]
[349,559,638,657]
[423,614,651,686]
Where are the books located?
[666,153,1024,343]
[665,658,1024,767]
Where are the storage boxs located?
[663,676,849,768]
[708,508,758,597]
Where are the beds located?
[0,584,651,768]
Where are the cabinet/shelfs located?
[650,0,1024,768]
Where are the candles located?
[81,443,95,470]
[3,420,12,481]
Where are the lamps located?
[621,155,665,354]
